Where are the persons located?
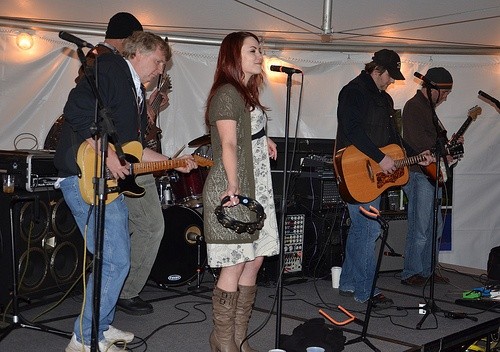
[204,32,280,352]
[56,32,197,352]
[76,11,198,316]
[334,49,433,312]
[401,67,464,285]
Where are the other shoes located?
[366,294,393,306]
[426,274,449,284]
[401,275,423,286]
[340,290,354,297]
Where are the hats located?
[105,12,142,39]
[373,49,405,80]
[422,66,453,89]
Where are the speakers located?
[0,193,89,308]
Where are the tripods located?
[395,88,479,329]
[339,220,389,352]
[0,207,74,342]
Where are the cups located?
[331,267,342,288]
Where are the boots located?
[235,284,260,352]
[209,285,240,352]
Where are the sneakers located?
[65,332,126,352]
[102,325,135,344]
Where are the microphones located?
[414,72,439,90]
[270,65,302,74]
[59,31,95,49]
[187,232,204,241]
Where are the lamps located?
[16,29,33,49]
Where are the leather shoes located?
[116,296,154,315]
